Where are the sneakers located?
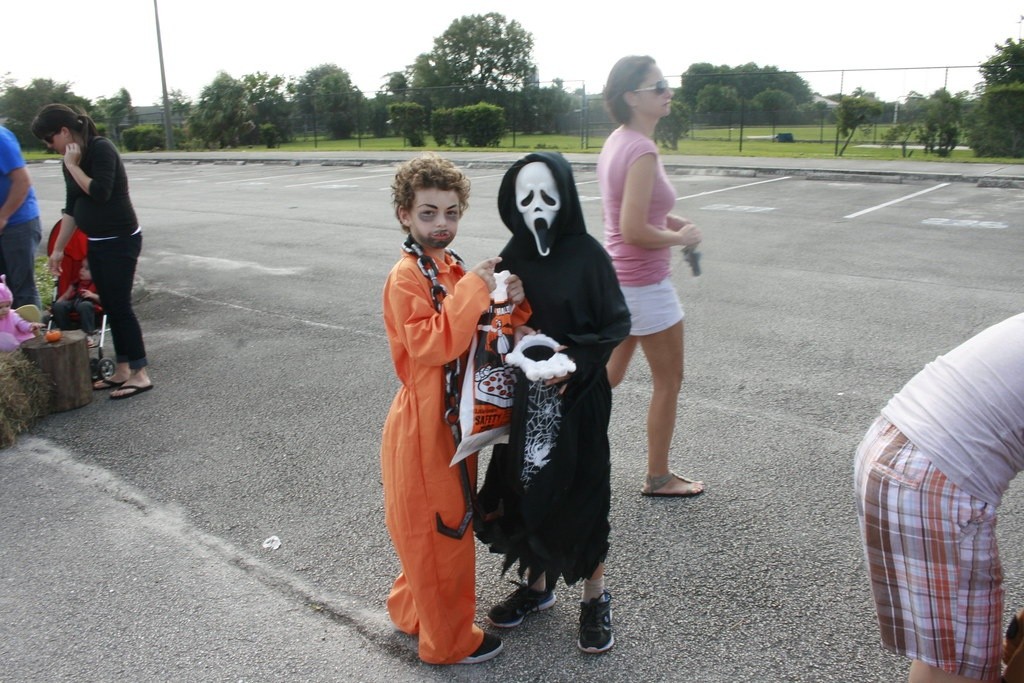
[489,580,556,628]
[578,589,614,653]
[455,633,504,663]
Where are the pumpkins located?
[45,329,62,342]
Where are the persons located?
[47,255,103,348]
[31,103,154,400]
[856,313,1024,683]
[0,274,46,351]
[472,151,632,653]
[596,55,704,498]
[0,126,42,311]
[381,153,532,664]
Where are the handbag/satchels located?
[449,270,519,467]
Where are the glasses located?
[43,132,57,146]
[633,79,668,96]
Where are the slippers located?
[94,376,125,390]
[109,385,153,400]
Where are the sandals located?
[641,472,703,497]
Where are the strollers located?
[46,218,116,381]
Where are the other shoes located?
[87,331,101,348]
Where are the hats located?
[0,274,13,302]
